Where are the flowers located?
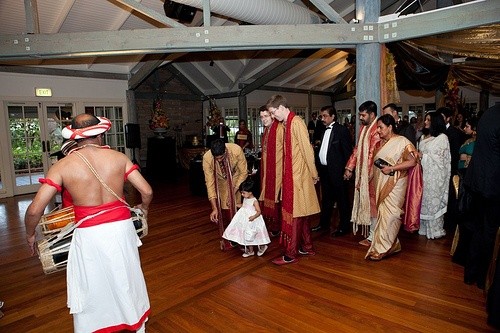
[205,95,225,129]
[440,79,467,112]
[150,95,167,133]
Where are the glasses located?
[269,108,277,116]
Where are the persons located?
[25,114,153,333]
[204,95,500,330]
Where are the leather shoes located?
[331,228,352,238]
[310,225,329,234]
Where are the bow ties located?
[325,127,331,130]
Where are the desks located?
[189,156,261,198]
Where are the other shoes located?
[358,238,372,247]
[256,245,268,256]
[242,251,254,257]
[271,255,295,265]
[369,252,387,260]
[296,248,315,256]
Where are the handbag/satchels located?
[374,158,395,176]
